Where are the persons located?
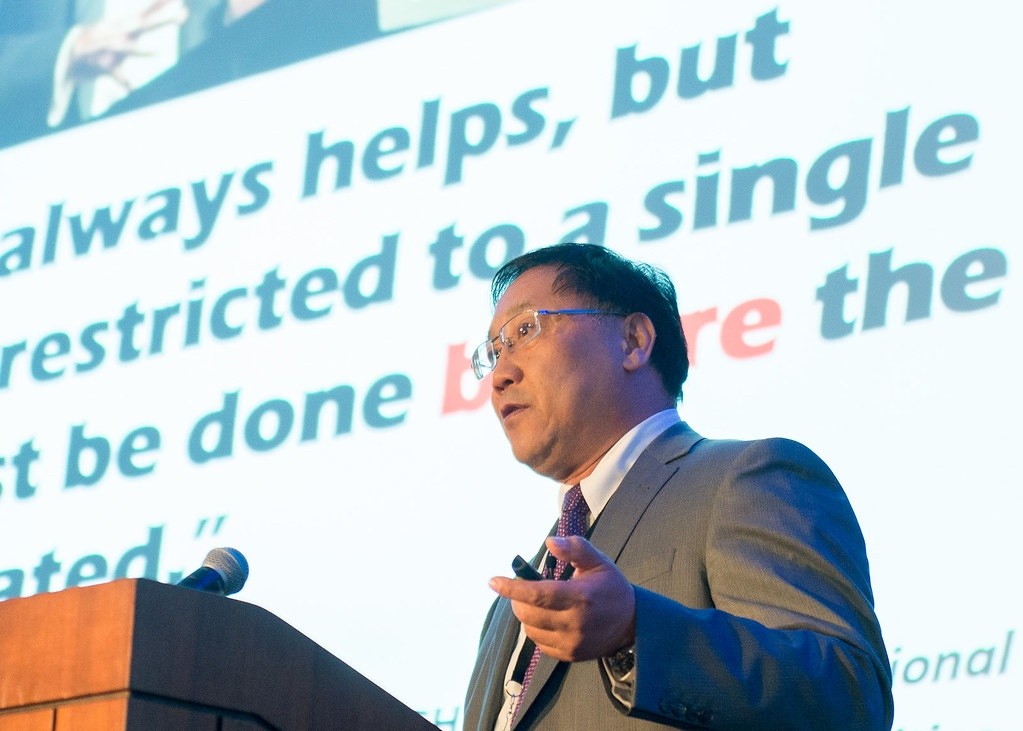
[461,242,894,731]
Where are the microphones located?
[174,547,250,599]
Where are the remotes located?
[512,555,546,580]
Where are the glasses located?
[470,309,633,381]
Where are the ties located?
[510,481,590,730]
